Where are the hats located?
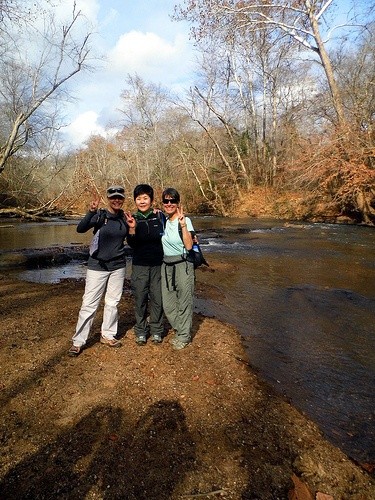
[106,186,126,198]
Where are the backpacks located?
[160,216,209,270]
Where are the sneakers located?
[169,330,193,350]
[151,334,162,345]
[68,346,81,356]
[136,335,147,346]
[99,334,122,347]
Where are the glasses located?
[107,188,125,194]
[162,199,177,204]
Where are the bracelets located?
[181,224,187,227]
[130,227,134,228]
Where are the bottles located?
[193,246,199,257]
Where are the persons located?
[153,188,194,350]
[124,184,166,346]
[67,186,129,356]
